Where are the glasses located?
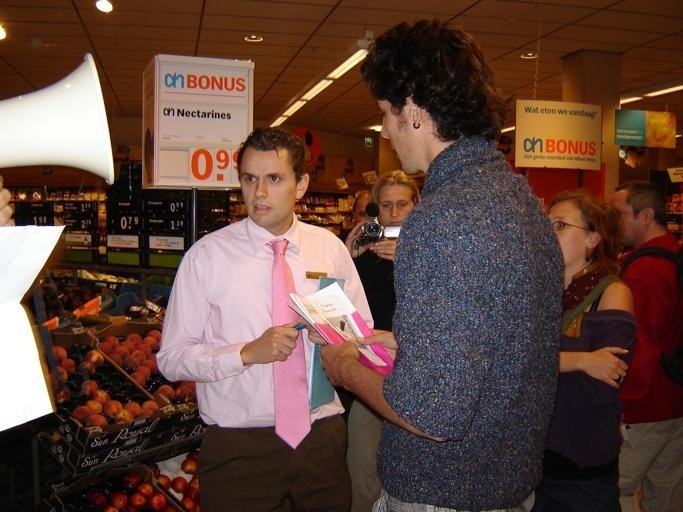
[551,220,590,232]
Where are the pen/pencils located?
[294,324,304,330]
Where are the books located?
[286,303,329,345]
[305,296,387,368]
[288,280,396,375]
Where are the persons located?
[535,189,635,512]
[0,171,18,226]
[612,180,681,512]
[338,190,372,245]
[154,125,376,510]
[319,13,563,511]
[343,168,419,510]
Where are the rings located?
[614,375,621,384]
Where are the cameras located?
[356,203,384,247]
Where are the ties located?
[267,237,312,451]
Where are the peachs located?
[51,329,199,512]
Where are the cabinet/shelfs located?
[297,190,354,230]
